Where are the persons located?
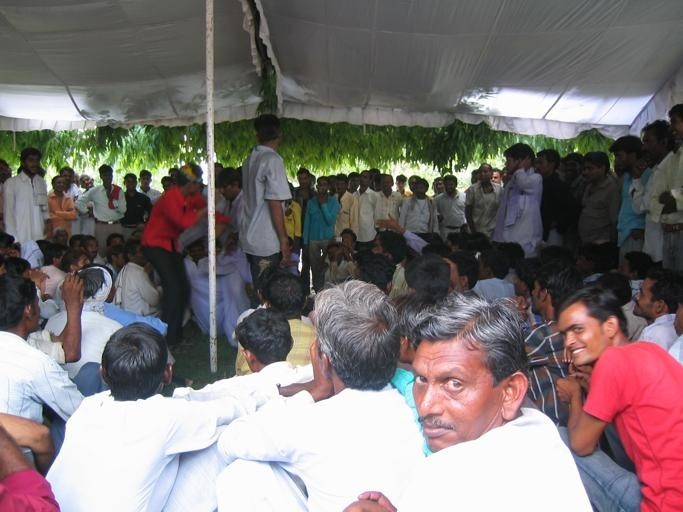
[0,254,86,365]
[136,170,161,204]
[629,120,675,263]
[501,242,525,284]
[537,148,572,248]
[575,240,605,284]
[46,176,76,241]
[596,274,649,341]
[632,268,683,372]
[214,162,226,214]
[63,249,88,272]
[70,235,81,250]
[46,322,315,512]
[38,242,67,297]
[59,166,81,235]
[370,231,409,298]
[80,235,104,267]
[343,291,592,512]
[408,175,421,196]
[0,413,56,477]
[405,253,449,300]
[171,308,314,403]
[491,168,502,185]
[375,214,474,257]
[502,167,510,184]
[324,227,358,268]
[471,170,478,184]
[216,167,243,247]
[53,227,69,244]
[303,176,339,293]
[565,153,587,252]
[80,174,94,235]
[369,168,381,191]
[73,174,78,187]
[667,273,683,366]
[104,245,127,283]
[616,251,652,298]
[493,143,542,261]
[465,164,503,239]
[5,257,59,330]
[334,174,358,243]
[649,103,683,270]
[510,261,586,427]
[514,257,546,330]
[609,135,653,266]
[215,281,433,512]
[42,267,122,378]
[347,172,359,193]
[0,231,20,260]
[0,274,101,470]
[232,266,283,347]
[75,164,126,257]
[553,286,683,512]
[432,177,443,198]
[578,151,620,272]
[328,176,338,197]
[353,170,380,255]
[443,253,480,298]
[390,293,437,366]
[361,255,396,295]
[0,423,61,512]
[168,168,179,184]
[434,175,465,244]
[235,273,316,376]
[114,239,165,317]
[294,167,316,297]
[21,240,51,268]
[4,148,49,243]
[122,174,152,242]
[324,243,359,288]
[472,247,515,303]
[162,177,170,190]
[377,174,404,240]
[239,114,293,308]
[0,159,9,232]
[103,233,124,263]
[281,183,301,255]
[396,175,413,200]
[80,264,168,337]
[399,179,439,233]
[140,163,230,346]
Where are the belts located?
[96,220,119,225]
[661,223,683,233]
[445,225,460,229]
